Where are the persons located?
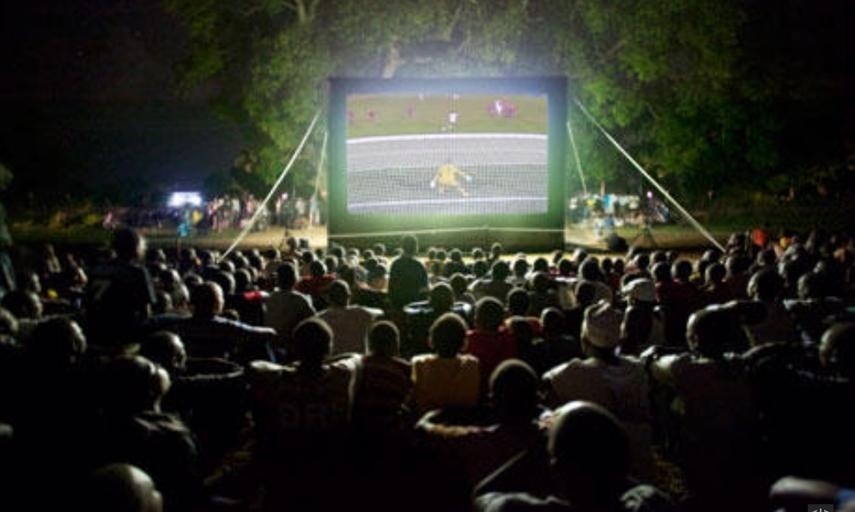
[485,97,518,118]
[405,103,414,118]
[367,110,377,121]
[0,187,854,512]
[430,161,472,197]
[348,110,357,127]
[447,110,460,132]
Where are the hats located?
[323,280,351,300]
[582,300,621,347]
[622,278,656,302]
